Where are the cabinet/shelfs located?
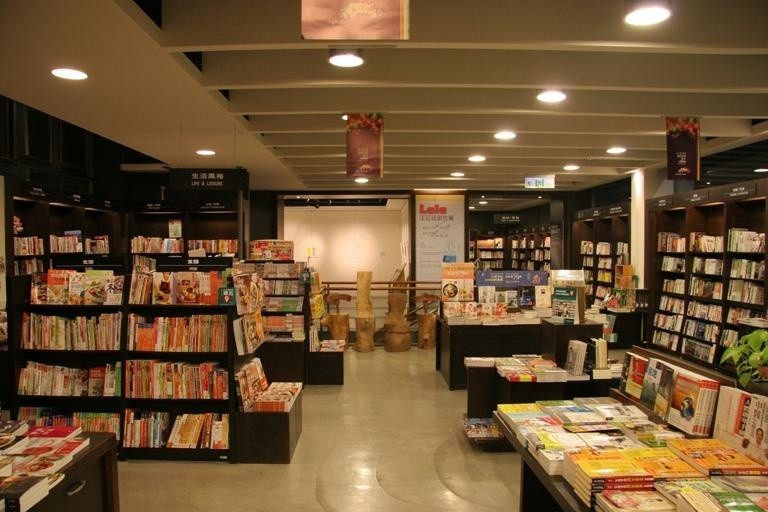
[467,183,767,375]
[0,169,347,512]
